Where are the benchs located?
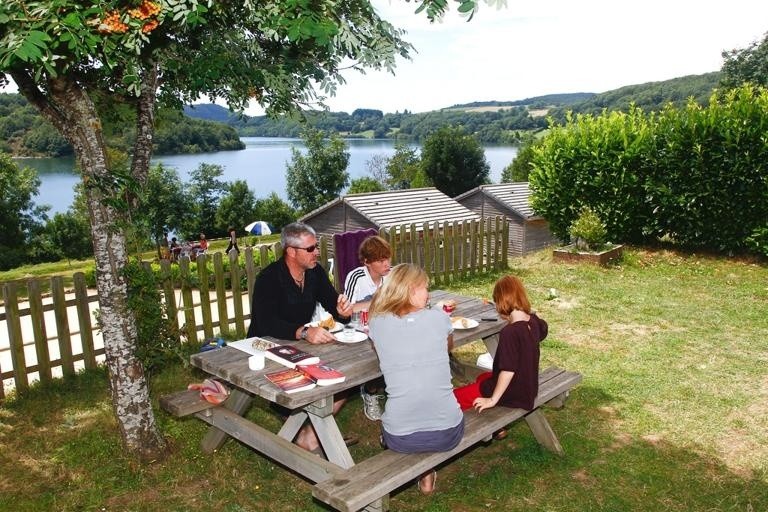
[310,363,582,512]
[159,380,233,419]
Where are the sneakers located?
[493,428,508,440]
[359,383,385,422]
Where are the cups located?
[247,354,265,370]
[351,306,362,324]
[427,294,432,309]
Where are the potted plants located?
[552,205,622,266]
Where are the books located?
[265,344,320,369]
[264,363,345,394]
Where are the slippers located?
[417,468,437,496]
[310,445,329,461]
[342,434,359,447]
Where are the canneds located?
[443,303,452,317]
[360,310,368,326]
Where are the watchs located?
[299,325,309,340]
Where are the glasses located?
[292,242,319,252]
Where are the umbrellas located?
[244,221,274,245]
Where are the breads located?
[318,316,335,329]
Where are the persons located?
[169,238,181,256]
[453,276,548,441]
[190,231,208,258]
[226,227,239,255]
[365,262,467,497]
[245,219,363,464]
[341,232,397,422]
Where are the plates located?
[331,330,368,345]
[445,316,479,330]
[347,319,369,331]
[304,320,345,333]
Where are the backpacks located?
[198,337,226,352]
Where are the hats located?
[187,377,229,405]
